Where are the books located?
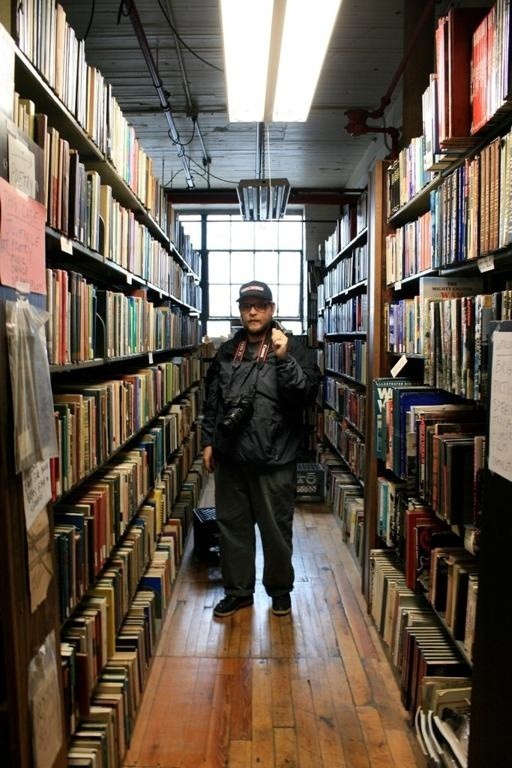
[1,0,213,767]
[306,0,511,768]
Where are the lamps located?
[220,0,341,123]
[235,124,291,223]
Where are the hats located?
[236,280,273,304]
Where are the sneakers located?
[211,592,253,617]
[271,589,292,615]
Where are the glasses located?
[239,302,268,313]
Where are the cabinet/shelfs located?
[306,76,511,598]
[0,1,210,762]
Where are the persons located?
[201,281,321,617]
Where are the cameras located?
[217,395,254,438]
